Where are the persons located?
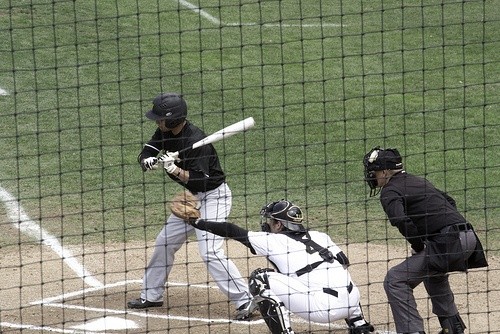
[363,146,489,334]
[170,194,374,334]
[126,93,260,320]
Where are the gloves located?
[141,157,158,171]
[158,151,178,174]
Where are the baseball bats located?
[140,116,255,172]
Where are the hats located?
[366,148,404,171]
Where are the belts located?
[440,223,471,233]
[191,188,210,196]
[323,281,353,298]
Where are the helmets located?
[265,200,306,232]
[145,91,188,129]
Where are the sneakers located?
[235,299,257,320]
[127,297,164,308]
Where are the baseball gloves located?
[169,194,202,222]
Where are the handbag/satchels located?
[469,228,488,272]
[423,229,466,273]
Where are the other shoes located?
[351,323,373,334]
